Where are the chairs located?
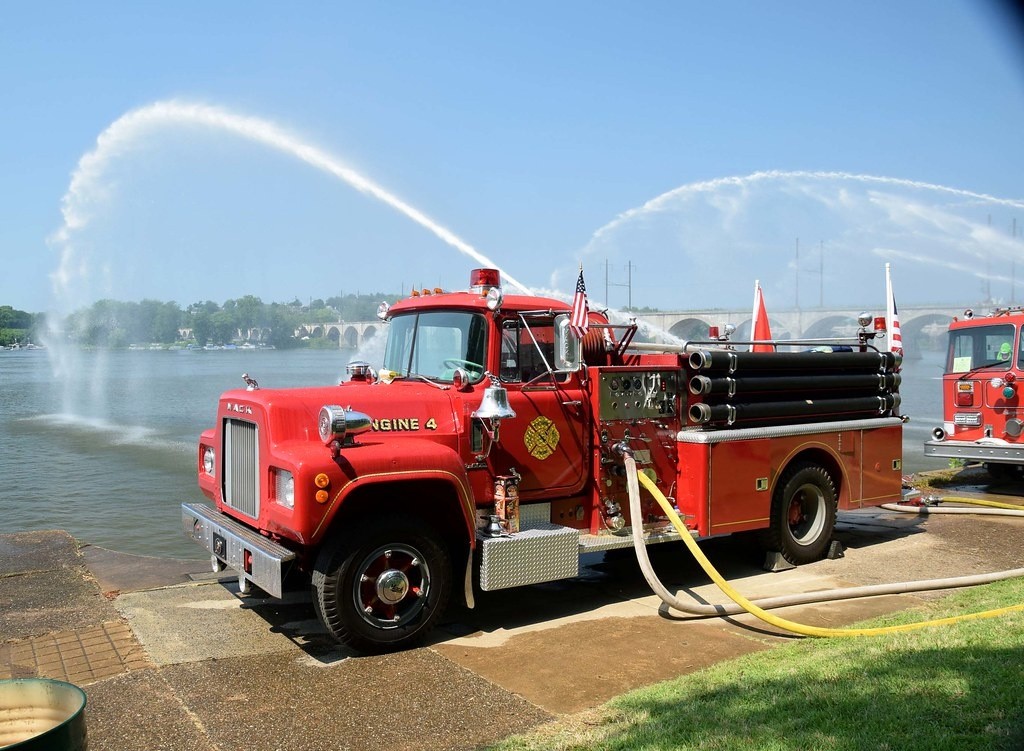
[527,341,553,382]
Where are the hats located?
[997,343,1012,361]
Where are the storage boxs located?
[494,475,519,532]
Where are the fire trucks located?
[923,303,1023,484]
[181,268,911,654]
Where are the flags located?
[754,288,774,352]
[570,271,589,339]
[889,282,903,354]
